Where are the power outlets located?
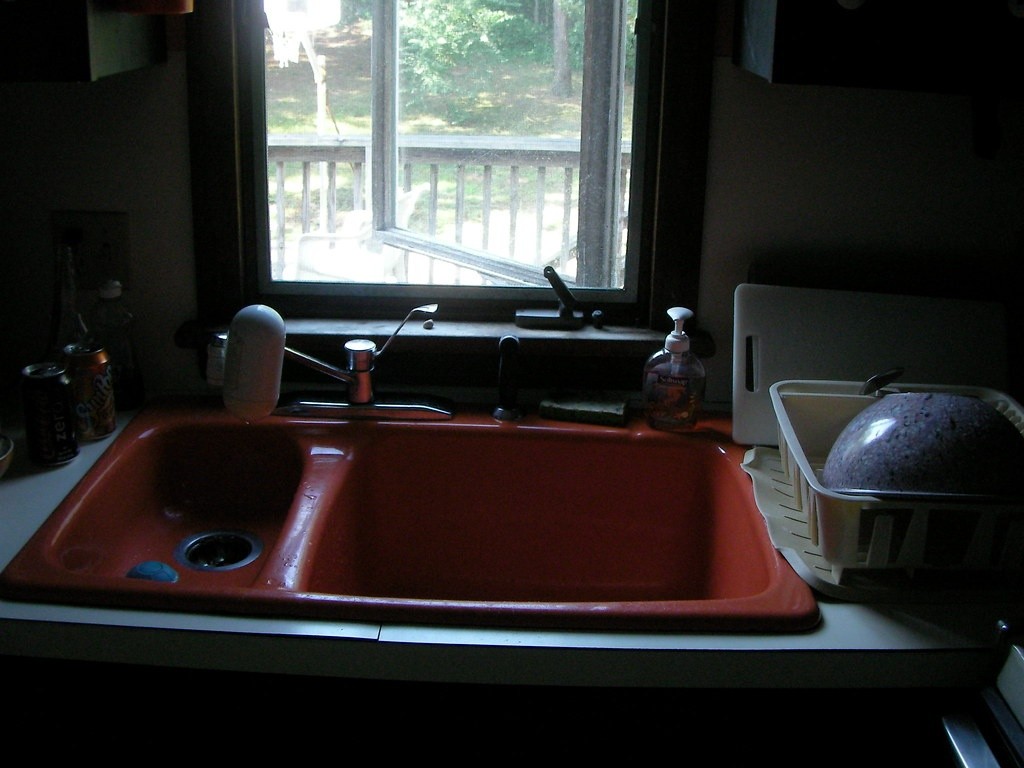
[50,209,137,294]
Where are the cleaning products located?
[640,304,707,429]
[88,278,136,406]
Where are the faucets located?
[222,299,440,423]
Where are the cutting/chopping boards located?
[731,281,1000,449]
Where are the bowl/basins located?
[0,432,15,479]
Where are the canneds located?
[19,361,82,468]
[60,341,118,442]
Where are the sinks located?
[287,418,822,636]
[0,394,315,617]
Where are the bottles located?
[205,333,230,387]
[58,279,146,414]
